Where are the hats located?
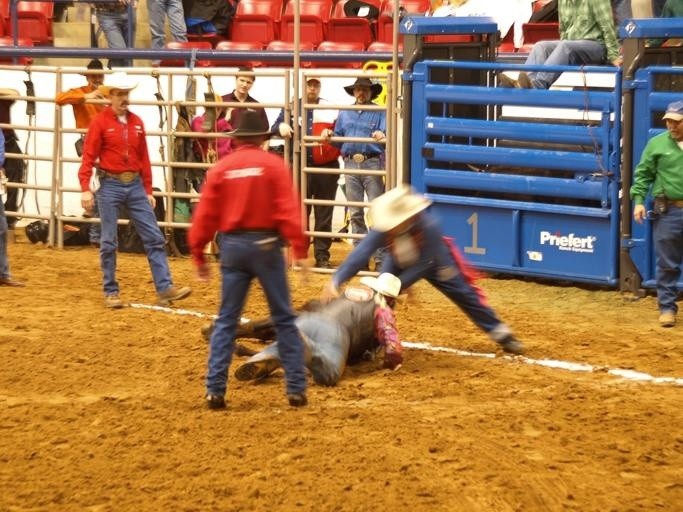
[661,99,683,123]
[224,110,275,142]
[343,76,384,98]
[359,273,408,300]
[362,182,435,234]
[306,76,322,84]
[96,70,139,97]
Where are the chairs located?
[398,2,557,64]
[162,0,393,70]
[0,0,108,69]
[132,0,152,69]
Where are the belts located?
[224,229,277,237]
[654,197,682,210]
[345,152,378,163]
[97,168,140,185]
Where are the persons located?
[628,100,682,328]
[495,1,623,90]
[0,125,26,289]
[77,71,192,309]
[268,76,341,271]
[189,93,235,192]
[0,86,28,231]
[185,109,310,411]
[146,1,189,67]
[233,271,403,388]
[320,185,527,357]
[318,76,387,271]
[217,66,268,150]
[53,59,107,248]
[92,1,137,67]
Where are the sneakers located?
[497,72,522,88]
[288,392,309,407]
[518,71,550,90]
[160,283,192,306]
[499,334,529,356]
[205,394,227,409]
[104,293,124,309]
[0,276,26,288]
[234,357,281,382]
[659,310,678,328]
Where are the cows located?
[466,138,601,207]
[201,297,397,363]
